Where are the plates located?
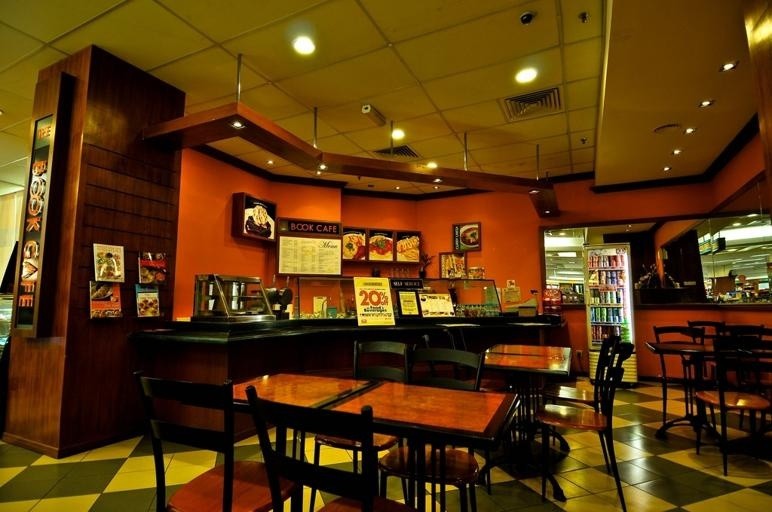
[244,208,275,240]
[343,231,419,262]
[91,285,113,300]
[459,224,478,248]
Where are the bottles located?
[543,284,579,304]
[448,268,456,289]
[453,303,500,317]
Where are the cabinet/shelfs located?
[232,192,278,243]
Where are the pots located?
[264,274,277,304]
[277,275,293,304]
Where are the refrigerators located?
[582,242,638,386]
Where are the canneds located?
[591,326,619,342]
[590,307,622,323]
[587,256,623,267]
[587,270,622,285]
[588,287,622,304]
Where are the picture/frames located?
[439,252,466,279]
[452,222,481,251]
[395,231,422,265]
[343,227,367,262]
[367,230,395,263]
[276,233,343,277]
